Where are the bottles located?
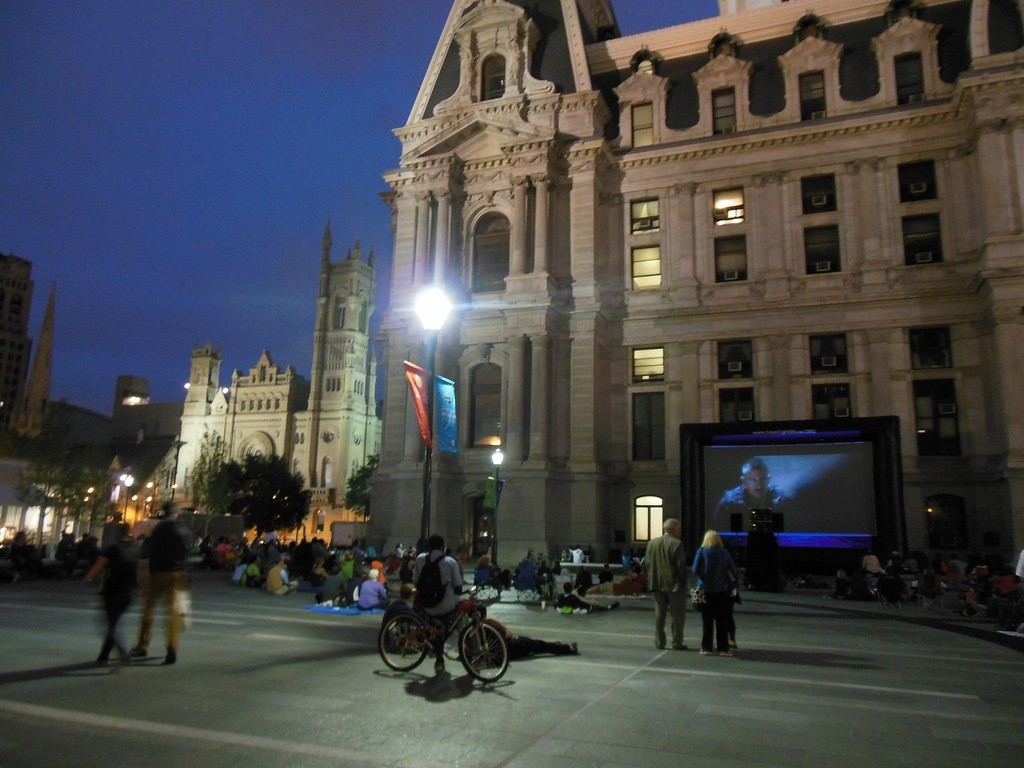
[561,550,567,562]
[432,624,445,636]
[567,551,572,562]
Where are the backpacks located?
[416,551,449,609]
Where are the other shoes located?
[699,638,738,657]
[130,648,147,657]
[164,645,177,663]
[673,644,689,651]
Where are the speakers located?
[614,530,626,544]
[730,514,743,533]
[983,531,1001,547]
[771,512,785,534]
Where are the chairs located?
[871,575,948,613]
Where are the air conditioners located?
[915,252,933,263]
[816,261,832,272]
[938,403,955,414]
[724,270,738,280]
[728,361,743,371]
[812,195,827,205]
[910,182,927,194]
[640,219,651,227]
[821,356,837,367]
[834,407,851,417]
[738,410,753,420]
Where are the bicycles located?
[377,583,512,684]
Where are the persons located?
[412,534,469,672]
[458,604,579,667]
[129,501,192,664]
[691,529,739,656]
[644,518,688,650]
[377,583,460,660]
[713,457,791,520]
[861,545,1024,599]
[0,528,642,613]
[83,522,138,669]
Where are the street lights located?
[123,475,135,522]
[490,448,503,564]
[412,283,455,548]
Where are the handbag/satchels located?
[246,574,264,589]
[690,584,710,612]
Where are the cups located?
[622,555,630,568]
[541,601,546,609]
